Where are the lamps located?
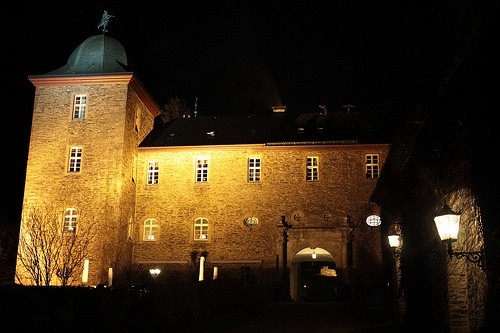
[433,199,486,273]
[388,228,409,260]
